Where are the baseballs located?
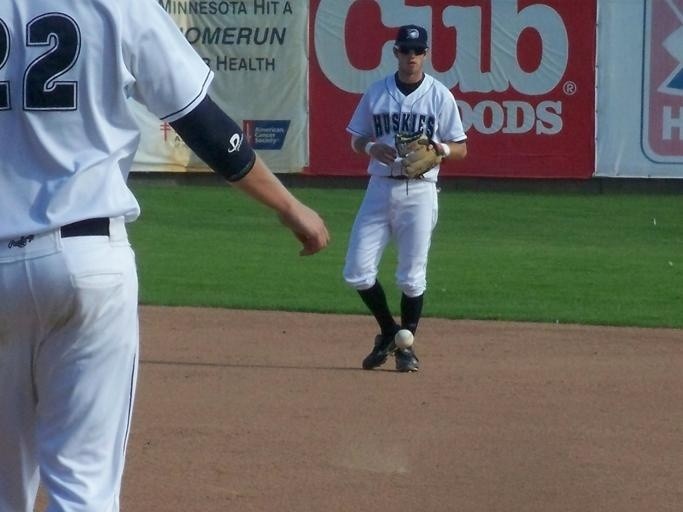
[395,329,413,348]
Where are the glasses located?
[396,46,426,56]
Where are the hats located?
[394,25,428,47]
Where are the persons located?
[343,25,469,372]
[0,0,334,512]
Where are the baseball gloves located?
[395,130,445,180]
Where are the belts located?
[61,217,111,238]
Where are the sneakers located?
[363,324,420,372]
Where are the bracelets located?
[441,142,450,156]
[365,142,376,155]
